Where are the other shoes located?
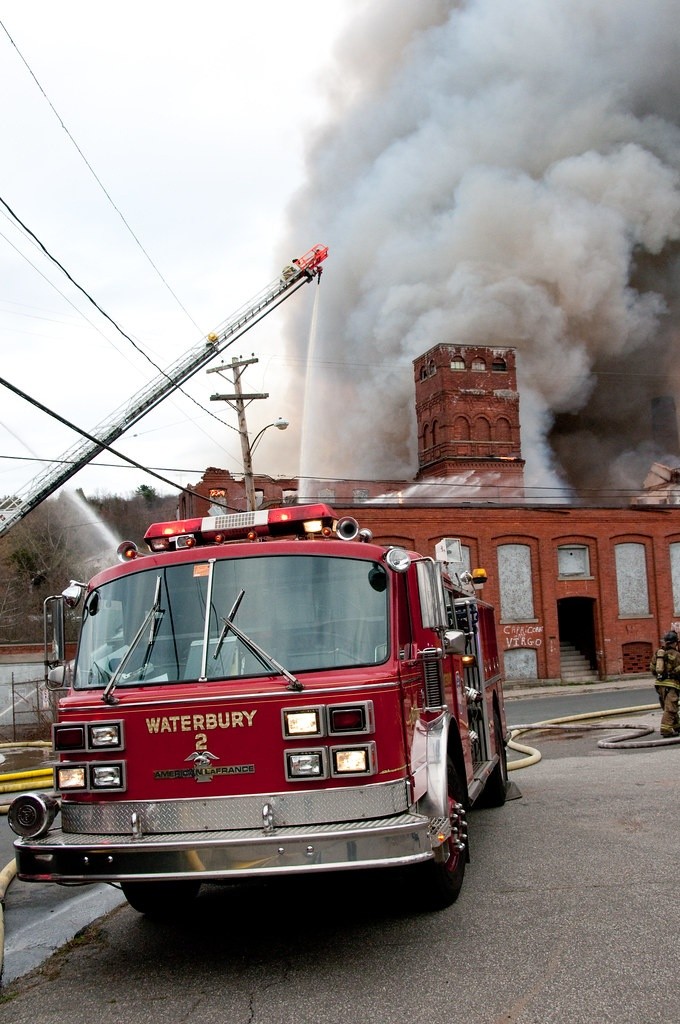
[663,732,678,738]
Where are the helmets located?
[665,632,678,642]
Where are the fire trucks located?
[7,503,512,916]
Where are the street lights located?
[244,416,289,513]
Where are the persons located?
[649,630,680,736]
